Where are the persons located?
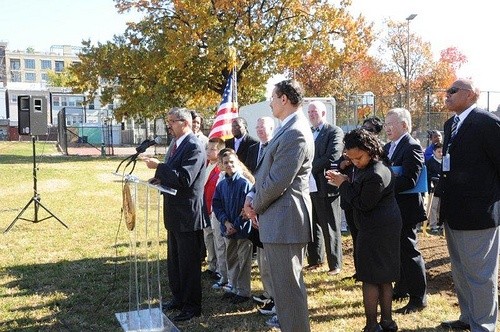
[434,79,500,332]
[190,80,443,332]
[141,108,208,321]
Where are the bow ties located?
[312,127,319,133]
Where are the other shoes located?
[265,315,279,325]
[223,292,237,298]
[253,294,268,302]
[257,299,276,314]
[441,320,470,329]
[395,301,427,313]
[327,269,340,275]
[224,284,232,291]
[211,281,228,288]
[362,319,398,332]
[305,264,319,269]
[201,269,213,275]
[231,295,249,304]
[431,227,438,232]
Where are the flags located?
[208,69,238,139]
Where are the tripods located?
[3,134,70,232]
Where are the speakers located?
[18,94,48,135]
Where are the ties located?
[450,117,460,142]
[258,144,265,163]
[388,142,395,160]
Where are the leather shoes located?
[157,300,179,309]
[168,308,201,321]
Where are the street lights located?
[406,14,418,110]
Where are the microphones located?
[130,137,162,161]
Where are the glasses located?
[447,87,466,94]
[166,119,183,123]
[382,122,395,129]
[269,97,279,101]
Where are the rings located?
[247,213,250,215]
